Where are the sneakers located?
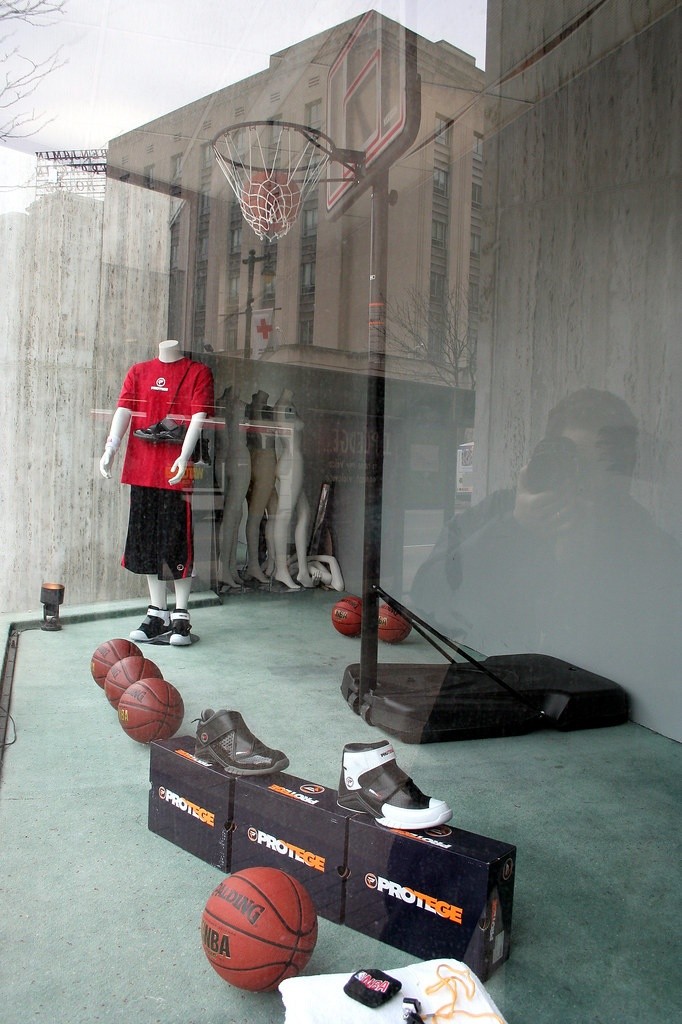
[130,605,173,641]
[192,439,211,468]
[339,741,453,829]
[168,608,191,646]
[189,708,290,776]
[132,416,186,443]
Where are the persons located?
[211,386,348,595]
[98,338,216,647]
[411,386,682,744]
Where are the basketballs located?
[378,603,413,643]
[117,678,184,745]
[332,596,362,637]
[90,638,144,690]
[104,656,164,711]
[201,867,318,994]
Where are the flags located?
[251,308,274,359]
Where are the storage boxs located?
[146,736,517,981]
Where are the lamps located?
[40,583,65,631]
